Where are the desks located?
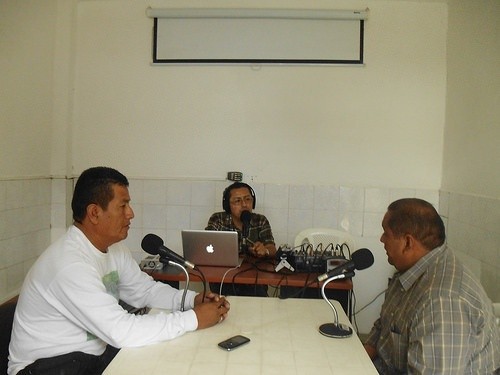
[101,295,380,375]
[151,262,353,315]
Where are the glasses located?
[230,195,252,206]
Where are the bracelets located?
[264,246,270,255]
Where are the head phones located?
[222,183,255,213]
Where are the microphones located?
[240,210,252,238]
[140,233,199,270]
[315,248,375,282]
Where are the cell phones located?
[218,335,250,351]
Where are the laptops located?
[181,230,245,266]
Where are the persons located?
[203,182,277,298]
[362,197,500,375]
[5,166,229,374]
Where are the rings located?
[218,316,224,324]
